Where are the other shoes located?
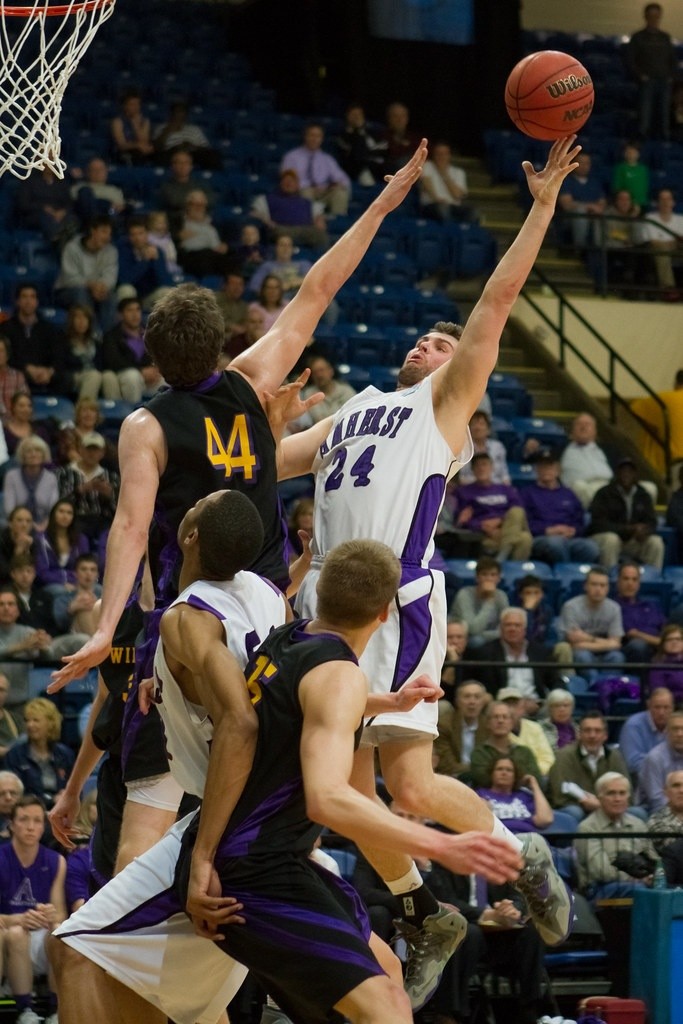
[18,1011,44,1024]
[47,1011,59,1024]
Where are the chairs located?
[0,13,683,999]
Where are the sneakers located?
[513,832,575,947]
[391,901,469,1014]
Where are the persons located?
[49,531,156,903]
[546,713,650,820]
[349,798,522,1024]
[0,769,25,844]
[172,538,523,1024]
[555,567,627,681]
[0,95,683,1024]
[467,606,570,708]
[50,218,120,332]
[486,878,577,1024]
[468,698,544,796]
[155,146,217,225]
[586,454,665,572]
[447,557,510,650]
[629,3,679,146]
[2,696,75,859]
[46,137,428,881]
[264,133,582,1009]
[47,488,296,1024]
[570,769,665,913]
[280,125,352,215]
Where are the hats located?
[83,430,106,451]
[470,452,490,463]
[497,688,523,703]
[527,446,556,464]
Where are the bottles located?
[469,973,512,1000]
[654,856,667,890]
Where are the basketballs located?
[505,48,596,143]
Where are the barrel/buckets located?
[579,995,646,1024]
